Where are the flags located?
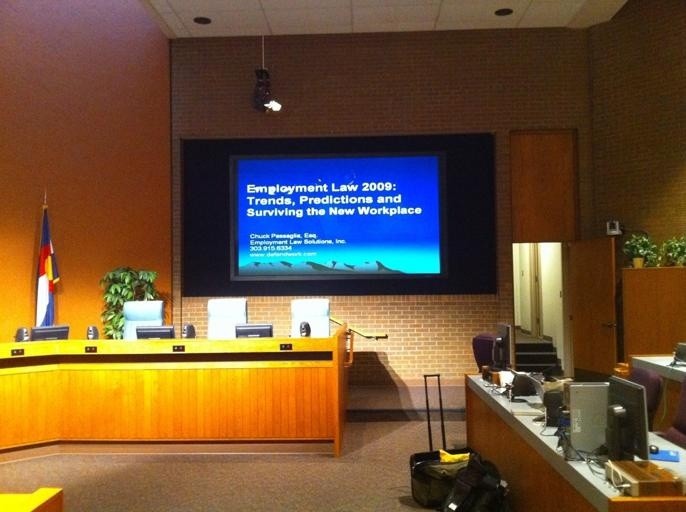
[34,203,61,327]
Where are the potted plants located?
[622,234,660,269]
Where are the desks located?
[466,371,686,510]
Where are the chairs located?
[122,299,330,341]
[625,368,686,450]
[472,333,508,371]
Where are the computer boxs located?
[563,381,610,454]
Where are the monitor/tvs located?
[605,375,649,461]
[235,324,273,337]
[491,323,512,372]
[228,151,449,282]
[135,325,175,339]
[31,326,69,340]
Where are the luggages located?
[410,374,474,508]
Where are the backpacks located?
[440,451,513,512]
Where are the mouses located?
[649,444,659,454]
